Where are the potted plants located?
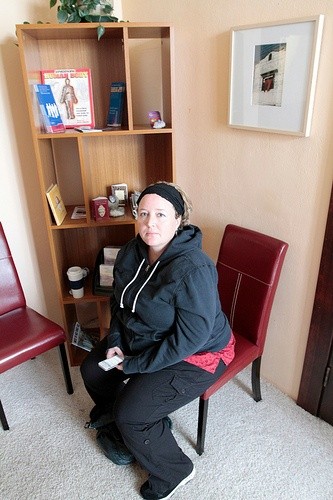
[24,0,127,41]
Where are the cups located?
[67,266,84,298]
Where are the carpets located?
[0,343,333,498]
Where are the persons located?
[79,179,236,500]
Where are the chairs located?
[195,225,289,457]
[0,222,75,432]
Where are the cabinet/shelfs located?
[15,23,175,368]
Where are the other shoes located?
[139,454,196,500]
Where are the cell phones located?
[98,354,124,371]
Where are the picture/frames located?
[227,13,325,138]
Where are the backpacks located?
[89,410,173,466]
